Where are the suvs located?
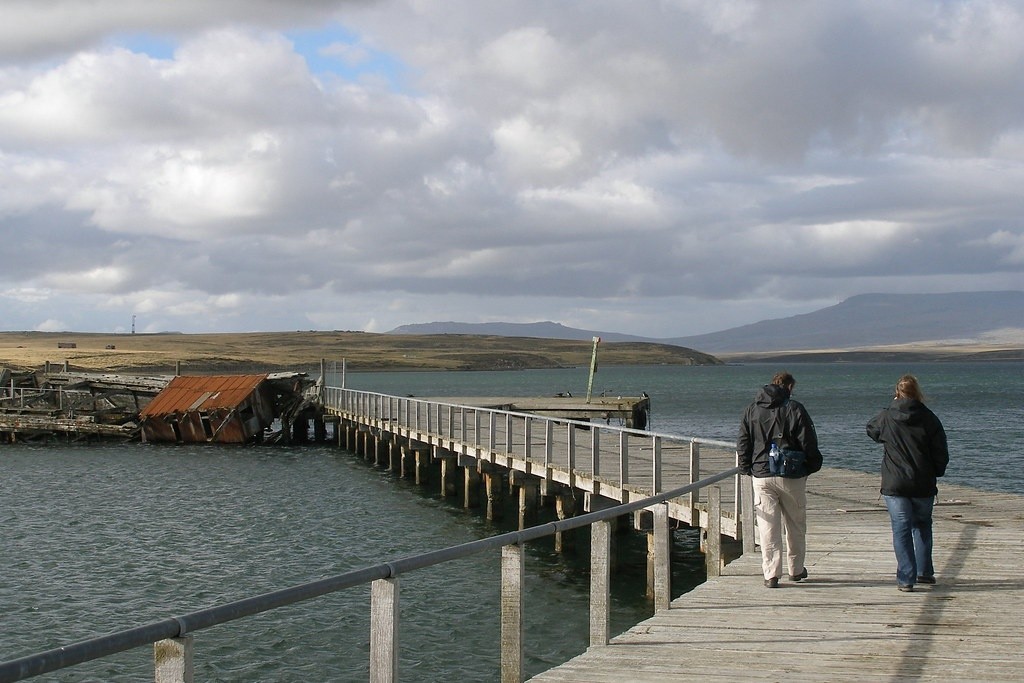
[105,344,115,350]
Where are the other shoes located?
[788,567,807,583]
[898,583,913,591]
[918,575,936,584]
[764,577,778,588]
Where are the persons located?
[737,374,823,588]
[866,375,949,592]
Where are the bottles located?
[769,444,779,473]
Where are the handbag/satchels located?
[769,402,806,479]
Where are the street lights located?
[586,337,601,404]
